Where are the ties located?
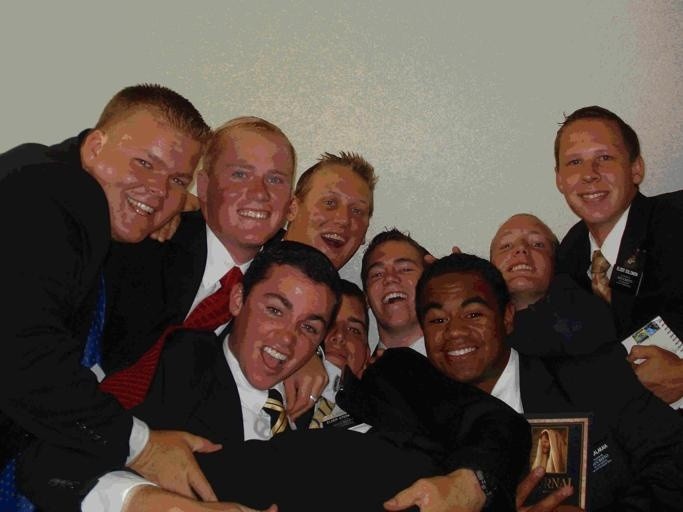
[262,389,291,435]
[98,266,244,410]
[1,263,107,512]
[309,396,336,429]
[590,250,611,304]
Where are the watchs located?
[474,472,494,499]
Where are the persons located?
[150,151,376,273]
[355,228,440,380]
[0,83,223,503]
[415,253,681,511]
[12,239,342,512]
[199,280,532,512]
[557,106,683,422]
[531,428,568,474]
[98,116,329,418]
[425,214,617,357]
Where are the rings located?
[310,395,317,403]
